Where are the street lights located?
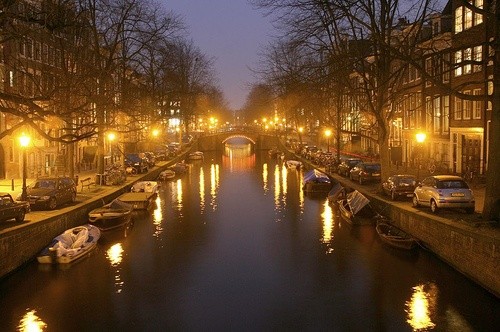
[108,132,116,165]
[19,135,30,202]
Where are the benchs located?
[80,176,96,191]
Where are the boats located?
[374,215,418,252]
[88,198,136,233]
[304,169,331,194]
[36,224,101,265]
[130,180,160,195]
[328,183,371,225]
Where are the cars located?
[15,177,78,210]
[412,174,476,215]
[286,137,342,173]
[348,162,381,185]
[123,141,205,181]
[381,174,418,201]
[337,158,364,178]
[0,192,31,224]
[286,159,304,171]
